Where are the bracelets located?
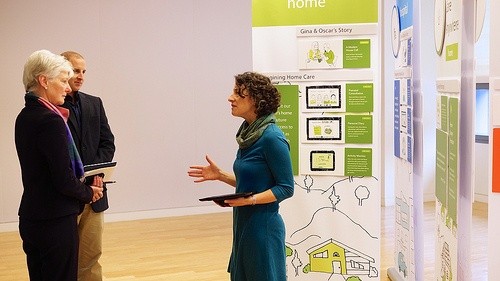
[250,195,257,206]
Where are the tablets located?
[199,192,254,201]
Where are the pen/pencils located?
[102,181,116,184]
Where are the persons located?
[41,51,115,281]
[187,72,295,281]
[15,50,104,281]
